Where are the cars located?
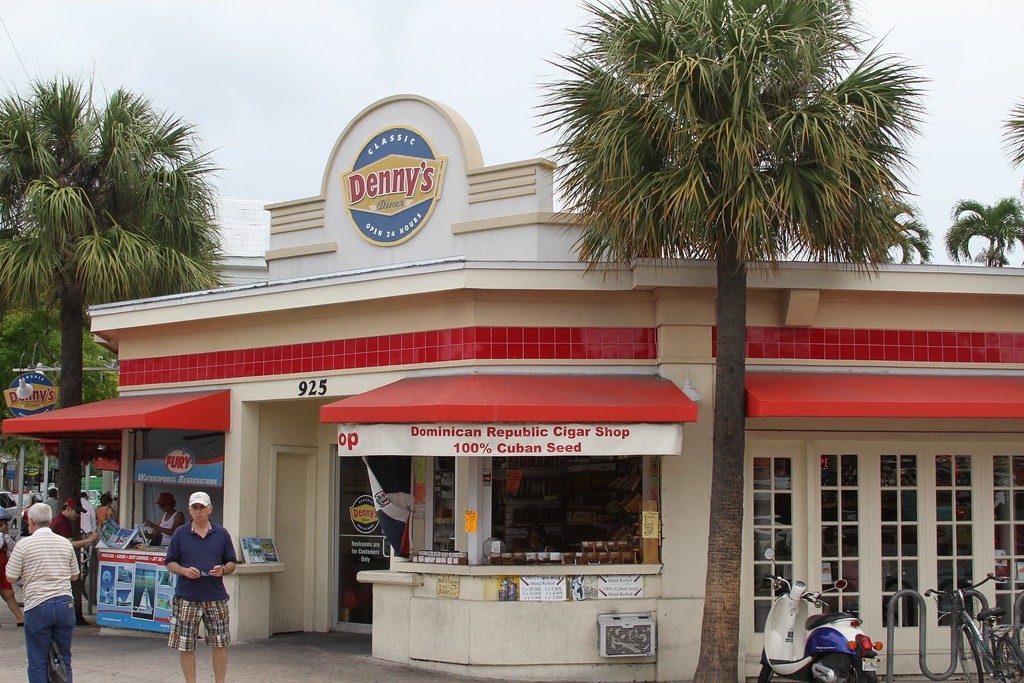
[0,490,102,528]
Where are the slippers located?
[17,623,25,626]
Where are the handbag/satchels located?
[48,641,68,683]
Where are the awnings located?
[0,388,232,440]
[319,374,700,455]
[743,368,1024,421]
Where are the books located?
[240,536,278,564]
[93,518,138,549]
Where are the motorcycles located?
[755,575,885,683]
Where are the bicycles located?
[925,572,1024,683]
[78,531,91,600]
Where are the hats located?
[154,493,176,505]
[189,491,211,507]
[63,498,87,513]
[0,507,13,522]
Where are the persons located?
[79,491,98,572]
[137,493,185,546]
[104,490,118,518]
[20,495,43,535]
[163,493,238,682]
[49,497,100,626]
[516,524,556,554]
[95,494,116,538]
[43,488,59,518]
[5,502,80,683]
[0,518,26,627]
